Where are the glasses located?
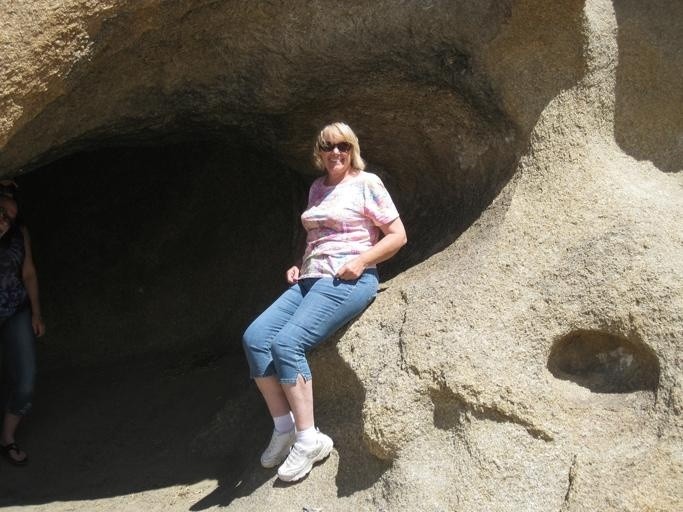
[320,141,351,152]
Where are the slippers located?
[1,442,31,465]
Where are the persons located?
[0,178,47,465]
[240,116,409,483]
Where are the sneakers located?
[260,425,296,469]
[277,430,333,482]
